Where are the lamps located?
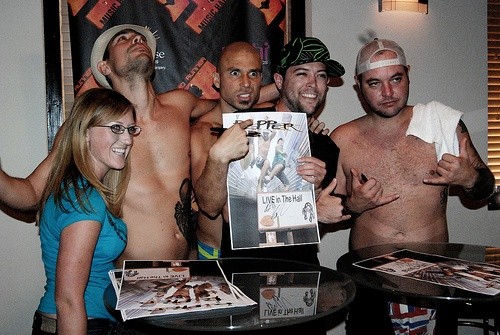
[379,0,428,15]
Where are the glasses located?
[89,124,142,136]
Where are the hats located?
[355,38,406,75]
[90,23,156,89]
[277,36,345,78]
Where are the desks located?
[104,242,500,335]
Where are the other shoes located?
[258,177,266,189]
[261,176,271,187]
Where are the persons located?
[189,41,326,260]
[140,280,232,314]
[330,39,495,335]
[220,35,340,267]
[32,88,142,335]
[0,23,280,266]
[238,120,289,194]
